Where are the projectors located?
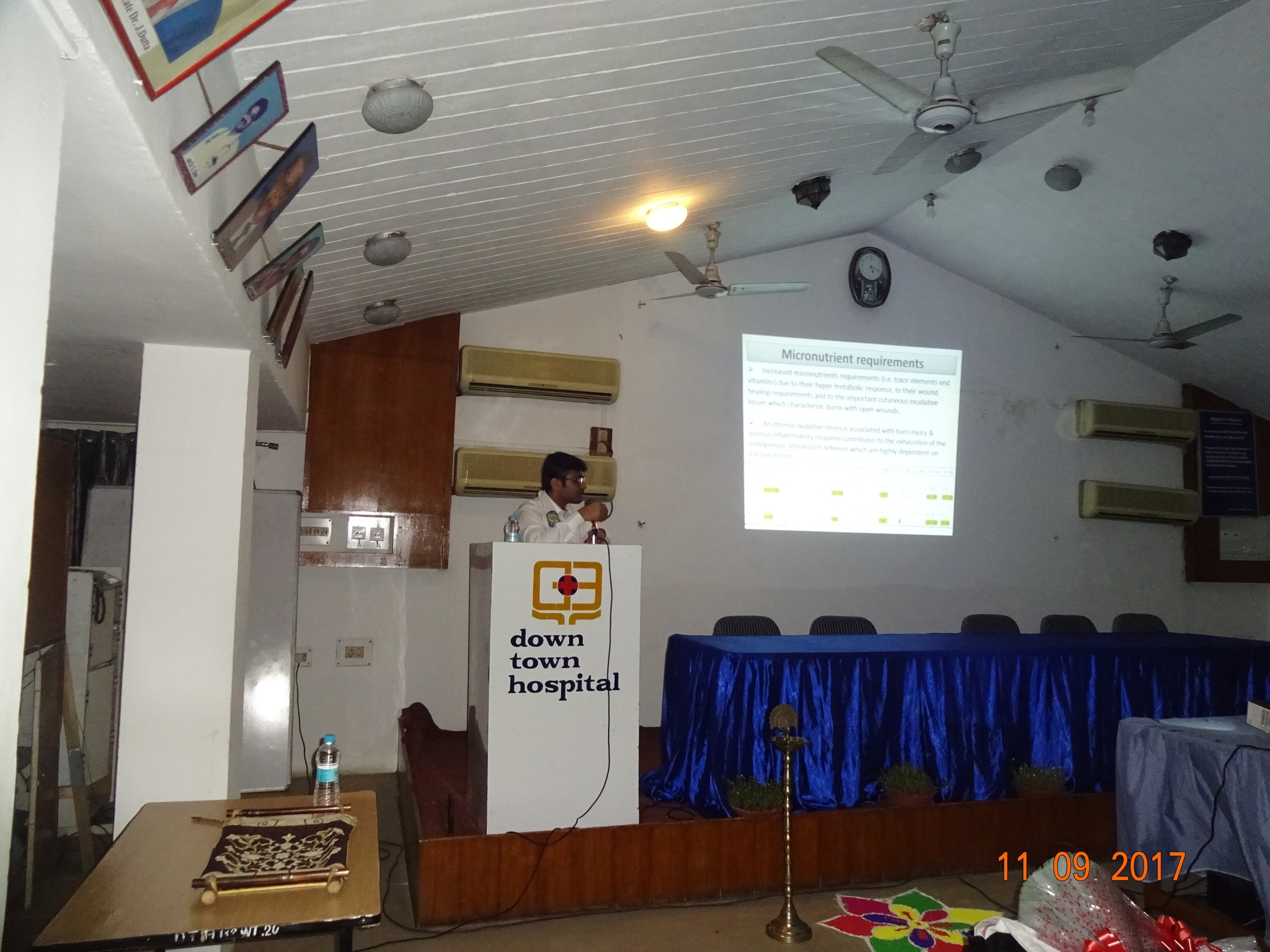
[1245,700,1269,733]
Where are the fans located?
[815,13,1128,176]
[648,222,810,301]
[1069,276,1243,350]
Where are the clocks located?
[848,246,892,308]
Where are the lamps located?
[791,176,831,210]
[924,193,937,218]
[1080,98,1098,127]
[1153,230,1193,261]
[1044,163,1082,191]
[944,148,982,174]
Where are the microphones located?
[585,498,599,535]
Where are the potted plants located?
[1011,757,1067,799]
[880,763,934,807]
[727,774,784,818]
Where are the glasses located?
[560,476,585,484]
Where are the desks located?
[1116,714,1270,952]
[642,631,1270,822]
[32,790,381,952]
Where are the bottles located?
[504,515,522,544]
[312,734,342,809]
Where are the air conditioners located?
[1075,398,1197,448]
[453,448,617,503]
[459,344,621,405]
[1078,479,1200,527]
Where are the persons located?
[182,98,269,188]
[513,451,608,545]
[141,0,224,65]
[216,151,313,270]
[247,237,320,300]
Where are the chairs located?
[1112,613,1169,632]
[712,615,781,636]
[960,614,1021,633]
[809,615,878,635]
[1040,614,1098,633]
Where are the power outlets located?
[335,637,374,666]
[294,647,312,666]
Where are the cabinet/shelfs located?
[55,564,122,827]
